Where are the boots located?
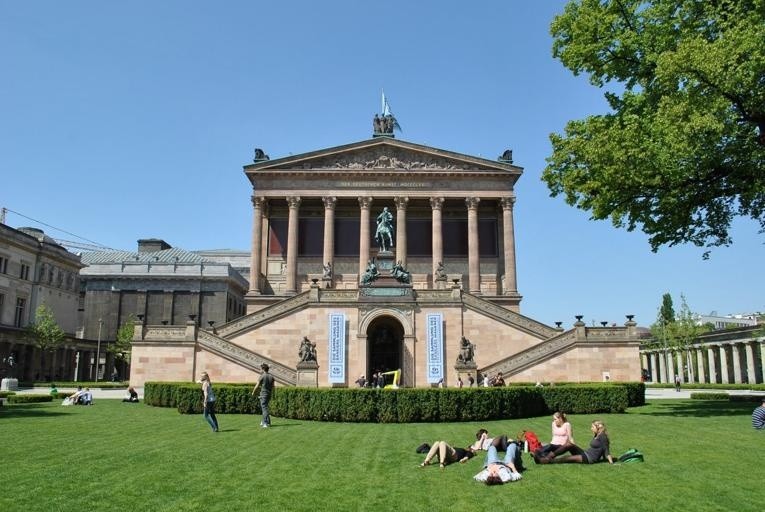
[530,448,556,464]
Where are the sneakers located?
[212,428,218,432]
[260,420,271,428]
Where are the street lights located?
[95,318,104,383]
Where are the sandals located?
[418,461,430,468]
[439,463,445,472]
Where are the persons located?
[474,442,526,487]
[434,261,448,280]
[456,377,464,388]
[467,372,474,387]
[380,113,388,133]
[418,440,476,469]
[252,363,275,428]
[68,384,83,405]
[438,378,444,388]
[73,387,93,405]
[460,335,472,364]
[323,259,336,280]
[376,205,395,247]
[297,335,316,361]
[530,411,575,457]
[471,428,509,453]
[675,374,682,392]
[360,259,379,283]
[491,371,506,386]
[364,373,377,389]
[377,372,384,388]
[6,352,16,377]
[533,419,614,465]
[200,370,219,431]
[484,374,489,387]
[518,429,542,454]
[752,399,765,430]
[122,386,139,402]
[386,114,397,133]
[393,259,409,282]
[373,113,380,132]
[354,375,365,388]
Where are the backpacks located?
[417,443,431,454]
[614,447,645,464]
[476,429,488,440]
[524,431,543,452]
